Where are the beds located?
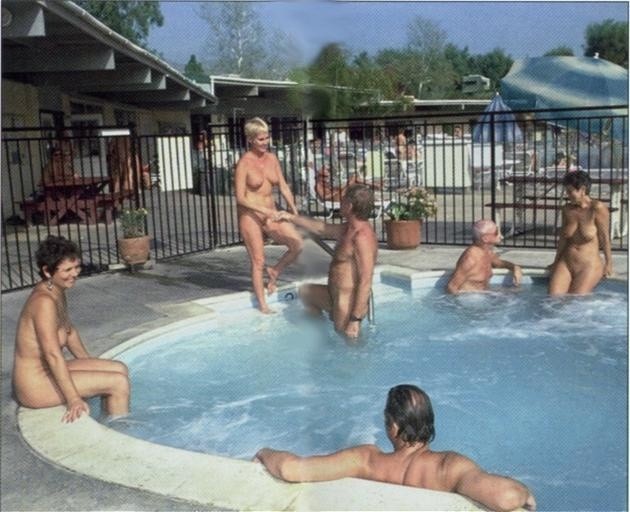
[472,92,523,142]
[499,51,629,147]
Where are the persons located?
[271,183,378,340]
[447,219,522,296]
[251,385,536,512]
[306,125,421,203]
[547,170,613,297]
[45,121,159,211]
[234,118,304,315]
[454,126,461,137]
[13,236,131,426]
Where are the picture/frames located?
[499,172,629,238]
[37,176,110,226]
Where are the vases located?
[116,206,149,239]
[382,186,440,221]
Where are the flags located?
[117,235,151,265]
[381,218,425,250]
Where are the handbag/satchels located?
[350,315,361,322]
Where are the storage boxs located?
[96,189,135,226]
[523,194,629,239]
[14,195,45,227]
[485,200,620,242]
